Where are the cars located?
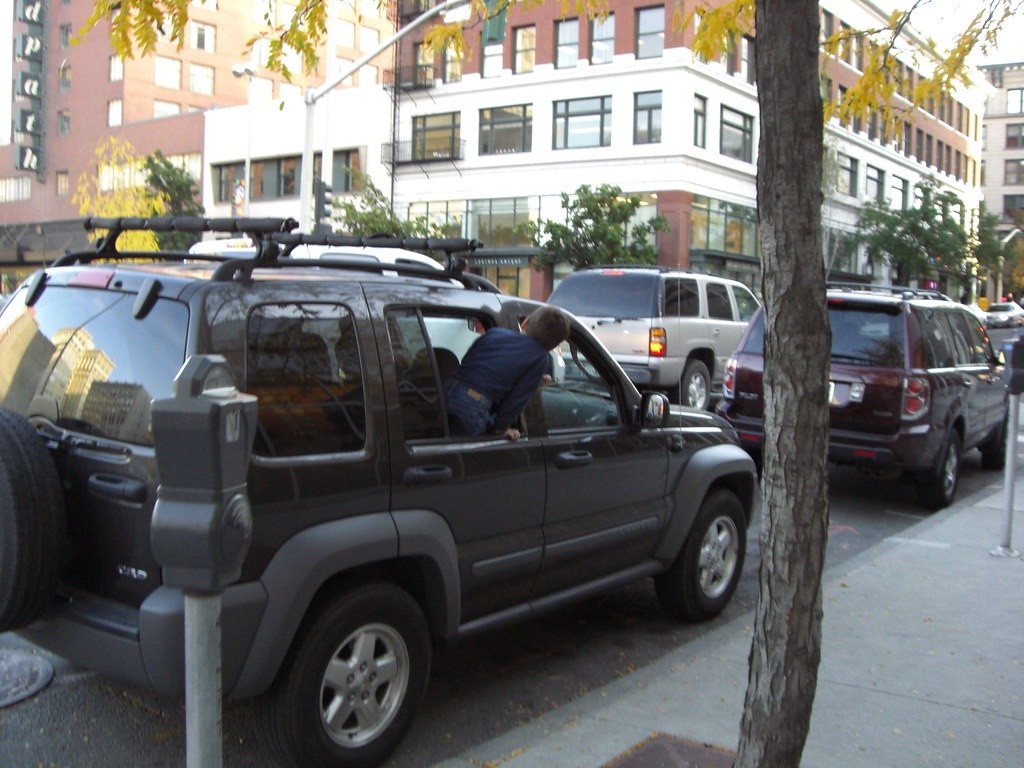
[987,300,1024,328]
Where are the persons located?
[1007,291,1021,304]
[440,305,571,440]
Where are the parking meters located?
[139,350,258,767]
[988,334,1023,560]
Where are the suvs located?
[712,279,1011,508]
[179,228,567,393]
[0,210,758,767]
[531,265,763,416]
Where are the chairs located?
[250,334,463,460]
